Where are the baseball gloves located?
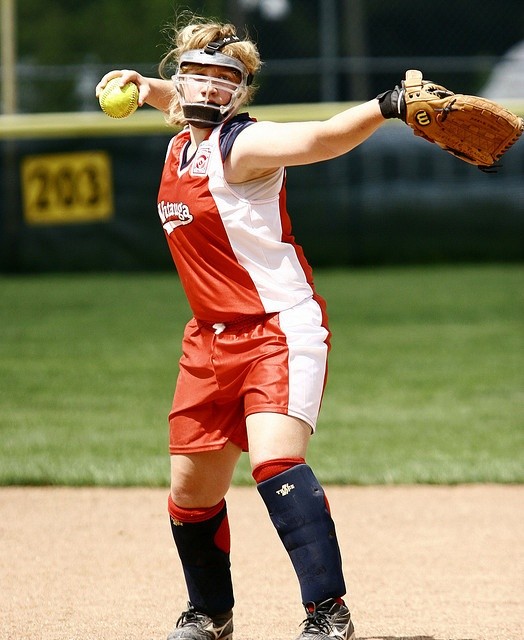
[376,70,524,173]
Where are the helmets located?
[171,36,252,130]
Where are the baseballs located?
[98,76,138,119]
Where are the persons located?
[96,20,524,636]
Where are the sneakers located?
[166,603,233,640]
[294,597,356,640]
